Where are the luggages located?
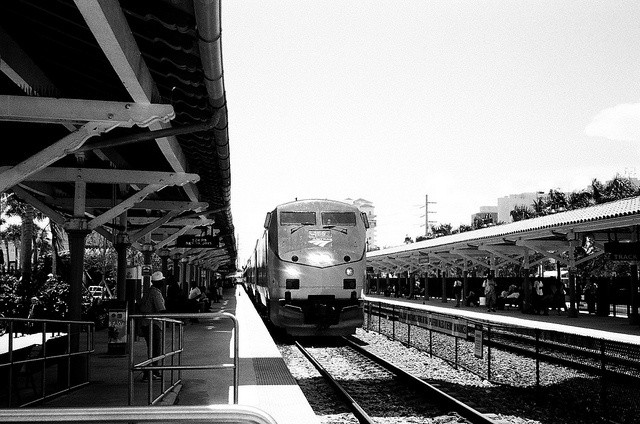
[200,298,204,313]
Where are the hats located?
[151,272,165,281]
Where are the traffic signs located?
[178,235,219,248]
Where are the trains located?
[243,198,370,337]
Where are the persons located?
[138,270,165,383]
[500,277,638,320]
[452,276,463,308]
[481,274,499,313]
[169,277,235,324]
[369,275,426,308]
